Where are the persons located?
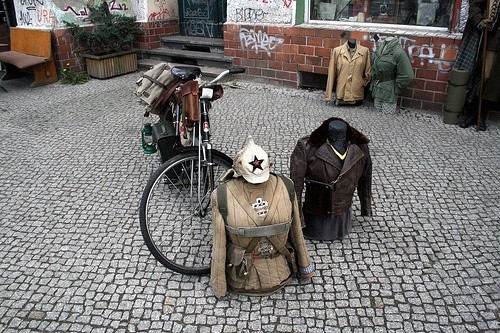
[370,37,412,114]
[462,1,500,131]
[290,118,372,240]
[210,146,314,300]
[323,38,370,104]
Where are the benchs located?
[0,27,60,88]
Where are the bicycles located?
[139,64,234,278]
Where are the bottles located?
[141,122,157,153]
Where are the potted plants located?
[63,1,141,80]
